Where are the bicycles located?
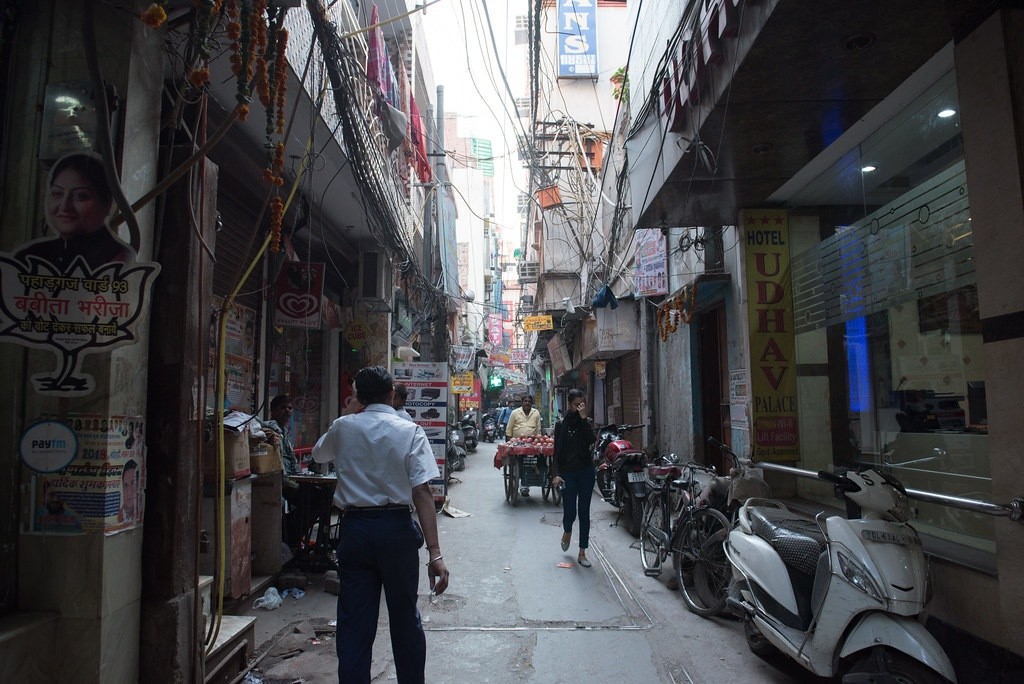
[629,454,737,616]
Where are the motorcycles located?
[670,436,755,536]
[592,424,650,539]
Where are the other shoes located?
[561,532,572,551]
[578,557,591,567]
[521,491,529,497]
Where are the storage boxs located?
[201,413,251,485]
[252,434,284,475]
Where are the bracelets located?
[426,555,443,568]
[425,544,440,551]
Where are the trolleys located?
[504,435,561,506]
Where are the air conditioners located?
[356,247,392,313]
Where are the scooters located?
[446,423,465,475]
[482,413,499,443]
[458,412,477,452]
[724,466,956,684]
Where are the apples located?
[507,433,555,448]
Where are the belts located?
[347,506,409,513]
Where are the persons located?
[551,389,596,567]
[117,460,141,522]
[68,419,143,449]
[312,364,450,684]
[17,154,131,265]
[505,394,542,496]
[269,396,322,545]
[393,384,413,422]
[497,401,515,430]
